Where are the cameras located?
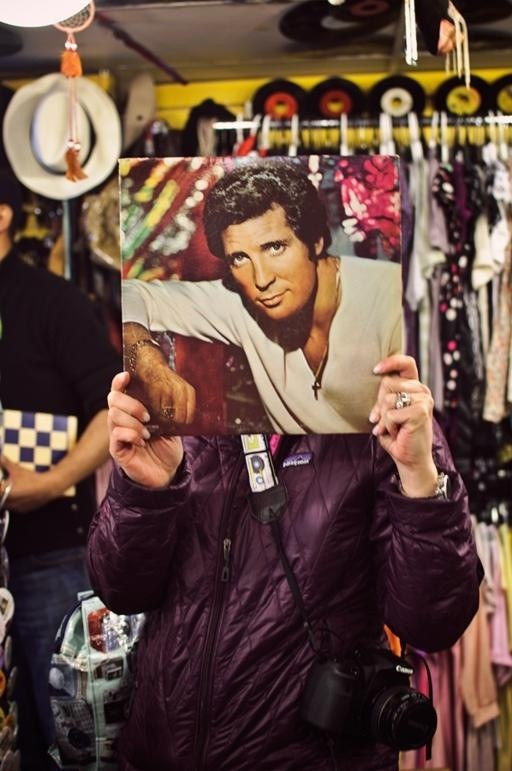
[301,646,437,751]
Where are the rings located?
[395,391,411,409]
[160,407,176,419]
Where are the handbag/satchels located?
[0,409,80,499]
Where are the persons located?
[0,169,122,771]
[85,352,484,771]
[121,167,407,435]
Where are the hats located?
[251,74,512,120]
[2,72,122,201]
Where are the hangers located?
[193,111,510,177]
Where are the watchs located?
[399,472,453,501]
[125,338,163,376]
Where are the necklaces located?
[311,258,342,400]
